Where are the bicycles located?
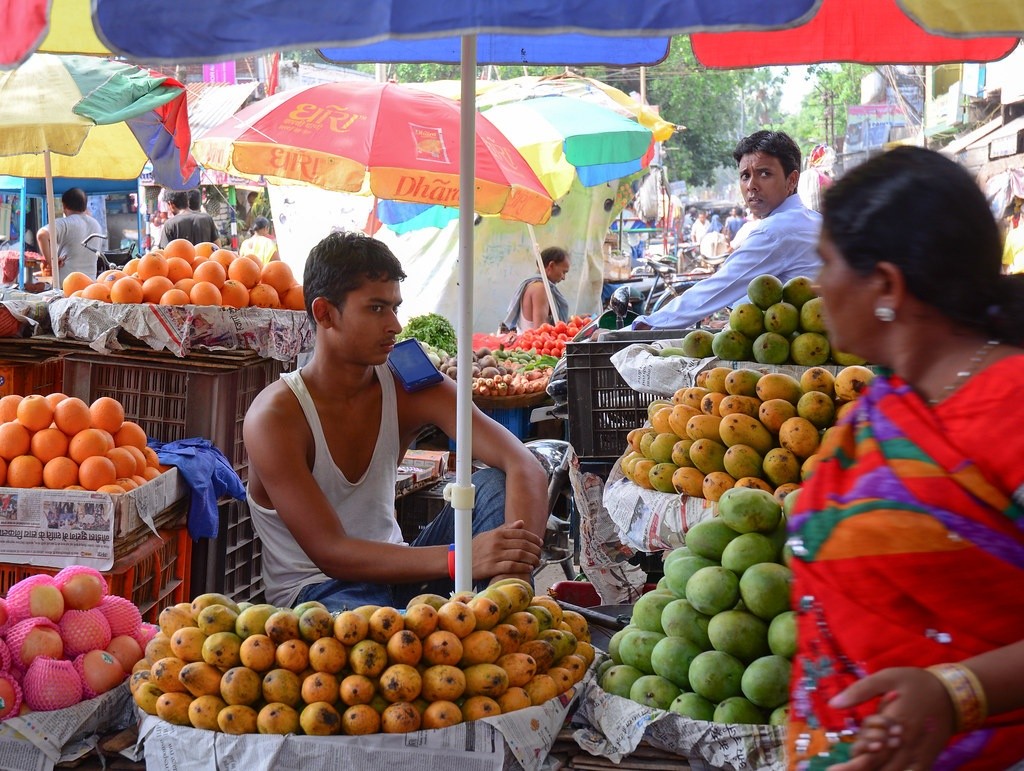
[604,259,698,317]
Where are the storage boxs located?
[0,352,298,626]
[396,330,723,573]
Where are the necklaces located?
[923,334,999,405]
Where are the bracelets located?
[925,659,988,732]
[446,544,457,580]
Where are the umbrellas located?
[0,0,1024,608]
[225,54,674,323]
[0,53,189,298]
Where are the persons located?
[37,188,101,292]
[784,147,1024,771]
[504,243,587,335]
[240,216,281,265]
[242,231,554,613]
[685,202,747,246]
[243,191,269,230]
[627,129,827,332]
[159,188,222,249]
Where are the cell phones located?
[385,337,444,392]
